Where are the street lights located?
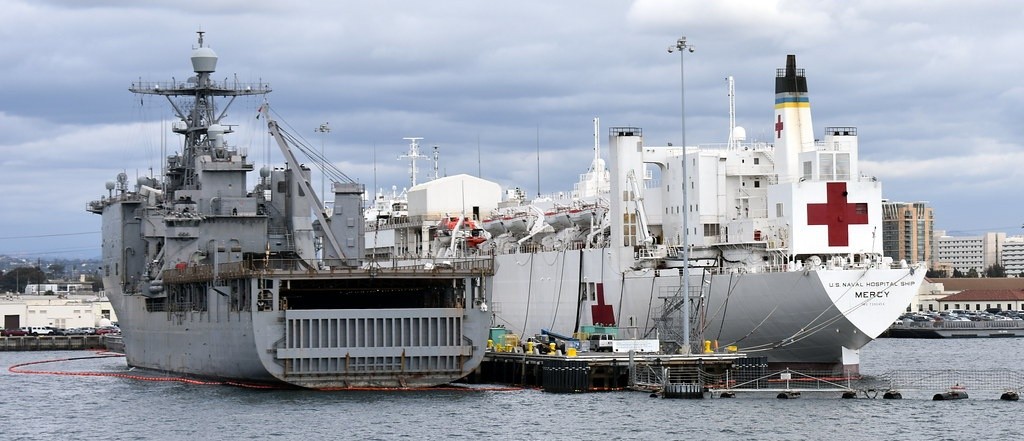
[668,37,695,355]
[315,122,330,203]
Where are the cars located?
[54,326,118,336]
[893,308,1024,325]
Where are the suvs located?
[4,328,28,337]
[588,334,614,352]
[31,326,53,336]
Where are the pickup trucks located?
[523,338,546,353]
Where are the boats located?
[86,25,497,390]
[314,55,928,378]
